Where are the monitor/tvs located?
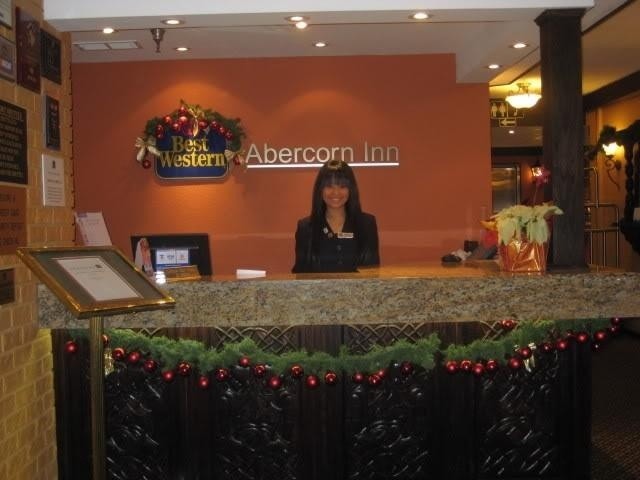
[131,233,212,275]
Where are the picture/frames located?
[0,0,67,207]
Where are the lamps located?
[505,82,542,110]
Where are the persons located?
[292,160,380,272]
